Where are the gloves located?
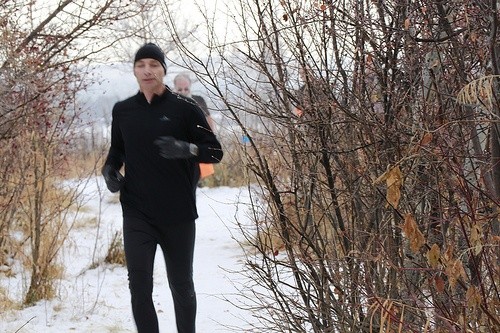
[102,165,124,193]
[154,136,197,161]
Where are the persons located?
[174,73,214,133]
[101,43,223,333]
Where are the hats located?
[134,42,167,75]
